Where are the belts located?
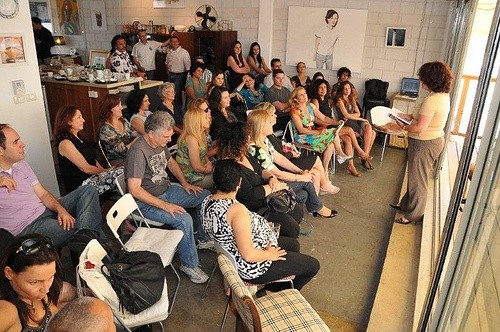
[169,71,186,75]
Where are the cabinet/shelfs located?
[152,30,237,72]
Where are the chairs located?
[288,121,336,175]
[370,106,407,161]
[362,79,390,118]
[106,193,184,315]
[201,243,295,332]
[115,173,164,228]
[217,253,331,332]
[79,239,169,332]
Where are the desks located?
[43,74,164,144]
[389,92,418,148]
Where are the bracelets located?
[295,174,297,182]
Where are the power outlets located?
[88,91,98,98]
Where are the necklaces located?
[26,301,34,312]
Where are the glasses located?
[6,236,55,266]
[205,107,210,113]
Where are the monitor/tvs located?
[401,78,421,93]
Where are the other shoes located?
[69,229,114,263]
[362,160,374,170]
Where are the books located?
[387,107,412,127]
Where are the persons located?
[313,10,339,69]
[392,29,396,46]
[388,61,453,225]
[0,30,377,332]
[31,16,58,66]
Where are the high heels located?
[336,154,353,165]
[396,217,417,224]
[389,203,403,212]
[356,151,373,161]
[346,166,362,177]
[313,206,338,218]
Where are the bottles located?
[95,9,102,26]
[170,25,174,33]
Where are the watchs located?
[403,125,407,131]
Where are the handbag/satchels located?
[257,187,296,220]
[101,250,165,314]
[281,122,301,154]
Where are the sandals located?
[320,181,340,195]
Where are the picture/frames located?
[385,27,407,48]
[88,49,108,65]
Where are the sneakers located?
[179,263,209,284]
[197,238,217,249]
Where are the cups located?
[111,72,130,80]
[89,74,93,83]
[48,72,53,78]
[96,70,102,79]
[67,69,73,75]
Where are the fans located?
[194,4,218,31]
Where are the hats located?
[273,69,283,75]
